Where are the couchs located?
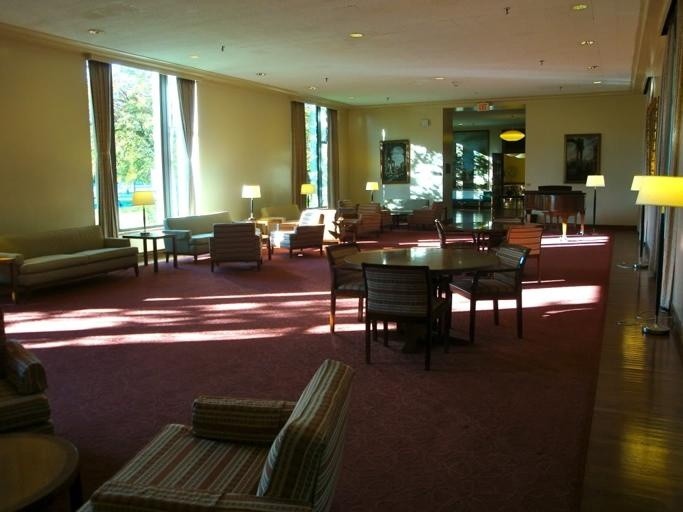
[261,204,301,230]
[0,225,140,302]
[163,212,239,262]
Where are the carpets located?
[1,226,617,511]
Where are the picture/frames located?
[563,133,601,184]
[380,140,410,185]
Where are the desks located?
[344,247,499,346]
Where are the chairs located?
[269,214,324,259]
[361,263,449,371]
[435,219,473,249]
[449,241,531,344]
[1,307,55,436]
[210,223,262,272]
[339,199,445,240]
[76,358,354,511]
[327,242,367,335]
[506,224,545,285]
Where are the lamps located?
[133,191,155,235]
[242,185,262,221]
[628,176,682,335]
[301,184,316,208]
[499,112,526,141]
[366,181,379,201]
[586,175,606,233]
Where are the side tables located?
[123,234,177,272]
[0,255,18,303]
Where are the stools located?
[544,211,577,229]
